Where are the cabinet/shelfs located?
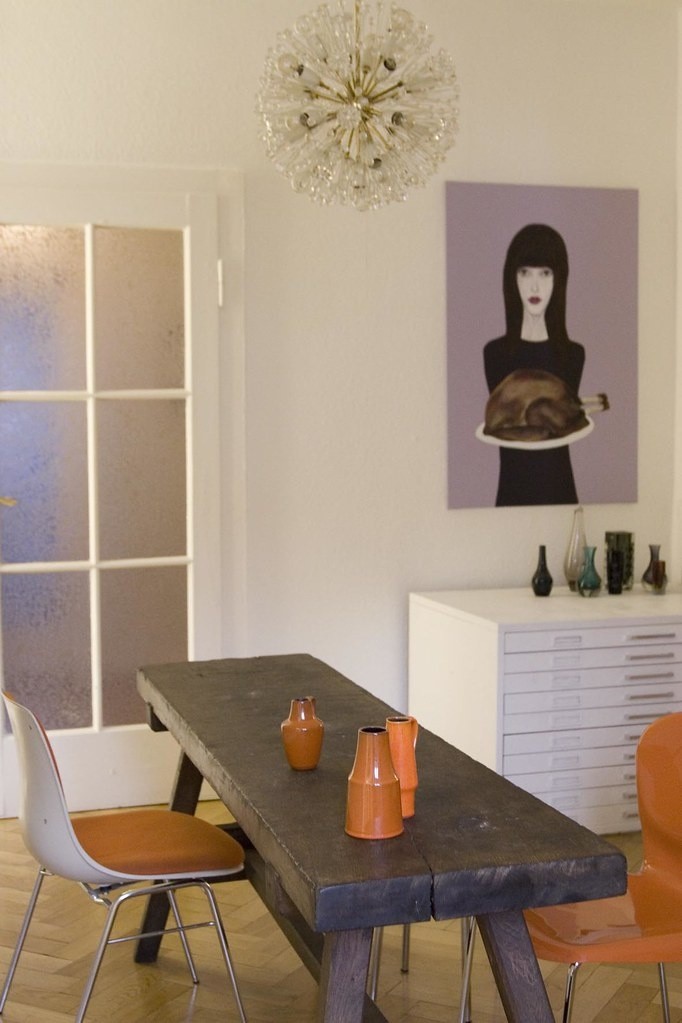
[407,582,682,837]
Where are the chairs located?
[0,688,248,1023]
[458,710,682,1023]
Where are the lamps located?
[254,0,462,213]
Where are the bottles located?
[385,716,418,819]
[642,545,669,595]
[281,696,324,771]
[531,546,552,596]
[577,547,601,598]
[344,726,405,839]
[562,509,588,592]
[609,550,622,593]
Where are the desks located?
[134,653,627,1023]
